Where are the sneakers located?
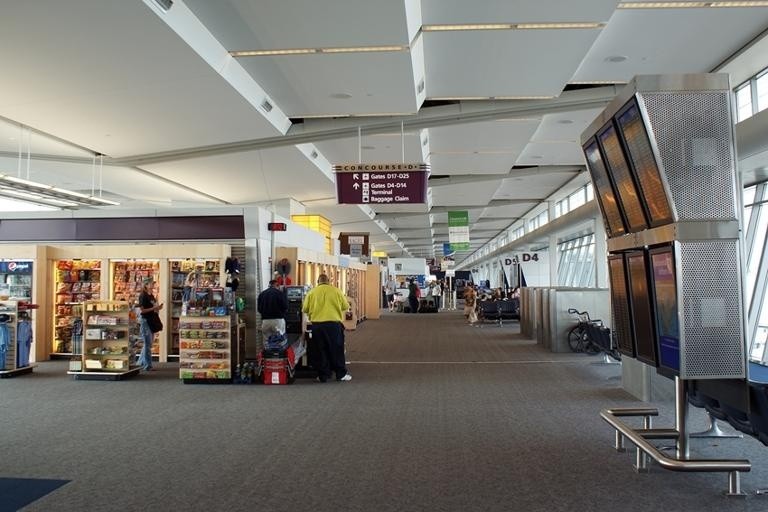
[336,374,352,381]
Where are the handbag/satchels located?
[468,311,477,323]
[148,312,163,332]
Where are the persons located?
[511,287,520,299]
[300,274,352,382]
[463,284,477,326]
[258,280,289,349]
[385,273,446,313]
[492,287,506,302]
[136,276,164,371]
[508,287,515,299]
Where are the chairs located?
[473,286,522,328]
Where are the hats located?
[144,278,155,284]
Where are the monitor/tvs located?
[285,286,304,301]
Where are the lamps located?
[0,123,120,210]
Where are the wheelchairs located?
[566,308,604,356]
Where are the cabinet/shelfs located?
[0,300,39,374]
[109,258,159,357]
[52,259,101,354]
[167,258,220,357]
[179,313,239,380]
[66,300,147,376]
